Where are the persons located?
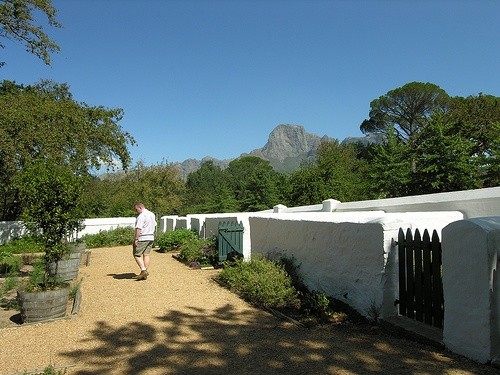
[132,203,158,281]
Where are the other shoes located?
[137,270,149,280]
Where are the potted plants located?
[29,156,81,280]
[17,243,70,323]
